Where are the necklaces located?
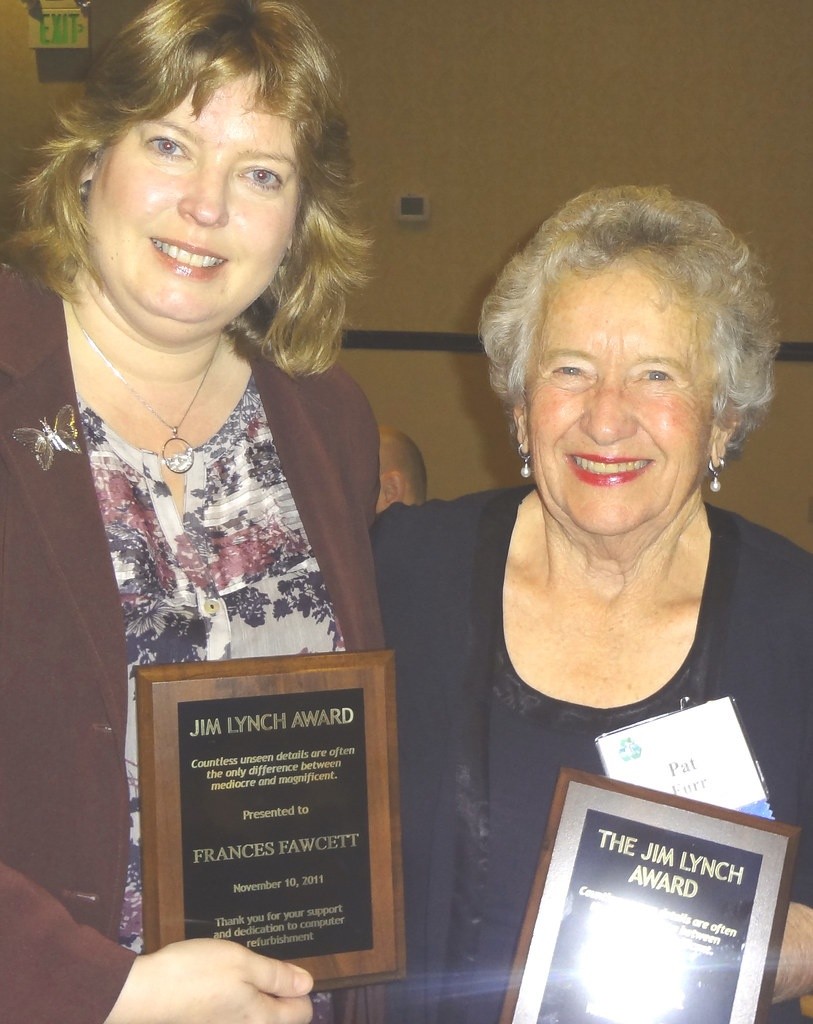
[68,297,221,473]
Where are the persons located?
[374,426,429,514]
[0,2,381,1024]
[364,185,813,1024]
